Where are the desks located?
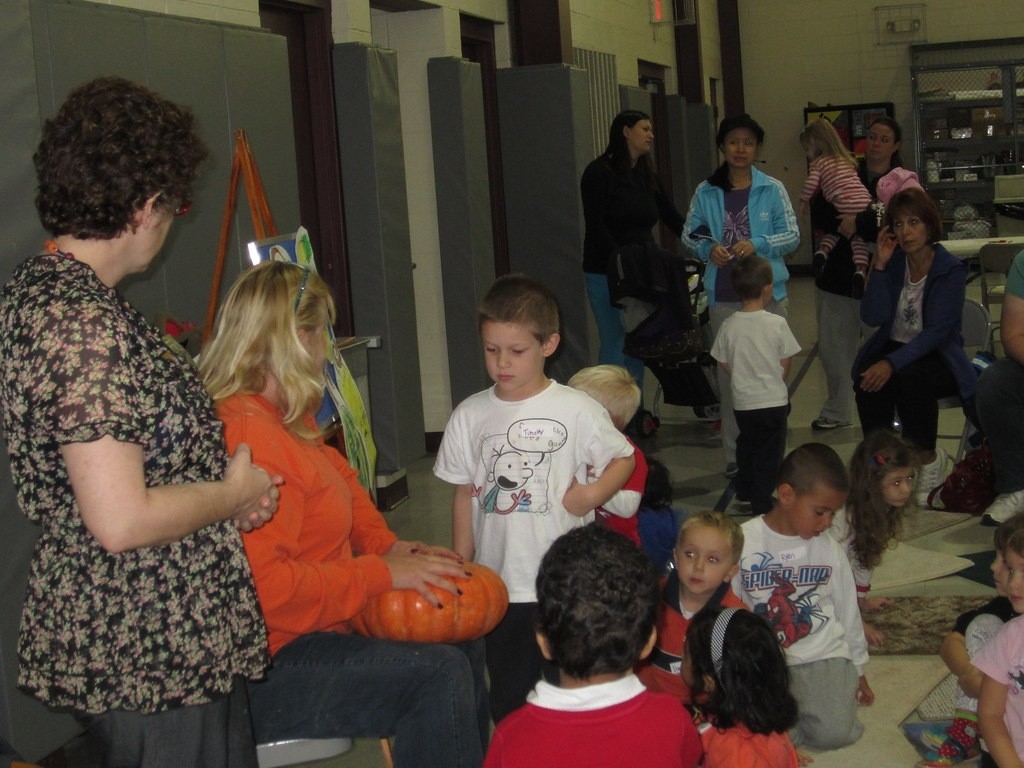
[938,235,1024,285]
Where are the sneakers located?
[915,448,946,509]
[811,416,852,430]
[981,488,1024,526]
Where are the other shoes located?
[915,734,984,768]
[812,249,827,276]
[852,271,867,294]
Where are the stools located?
[254,736,395,768]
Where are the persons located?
[849,186,977,505]
[196,259,493,768]
[635,509,751,728]
[567,364,682,566]
[0,74,285,768]
[972,248,1024,526]
[826,434,916,649]
[729,443,873,749]
[431,276,648,727]
[579,110,661,440]
[799,117,927,429]
[483,522,706,768]
[681,115,803,478]
[914,512,1024,768]
[677,604,800,768]
[710,254,803,516]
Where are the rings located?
[740,250,744,254]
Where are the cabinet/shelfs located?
[912,95,1024,213]
[803,102,896,253]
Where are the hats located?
[716,109,764,148]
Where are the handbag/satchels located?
[926,446,998,516]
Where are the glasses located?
[286,260,311,311]
[160,180,193,215]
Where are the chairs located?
[890,297,992,474]
[978,242,1024,344]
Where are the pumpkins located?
[349,563,509,643]
